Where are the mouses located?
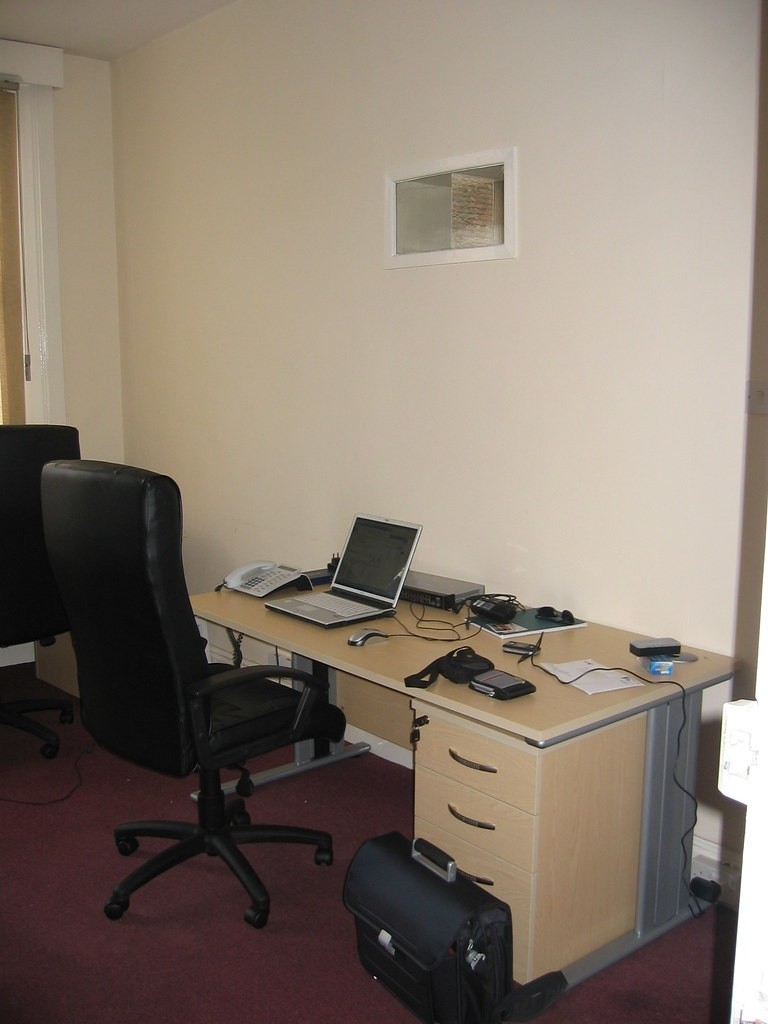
[348,627,389,647]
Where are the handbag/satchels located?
[342,830,569,1024]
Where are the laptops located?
[264,511,423,630]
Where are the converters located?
[471,598,513,623]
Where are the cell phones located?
[503,641,541,656]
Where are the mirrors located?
[383,145,519,272]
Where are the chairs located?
[0,425,81,761]
[39,458,347,927]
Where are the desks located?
[188,568,745,1024]
